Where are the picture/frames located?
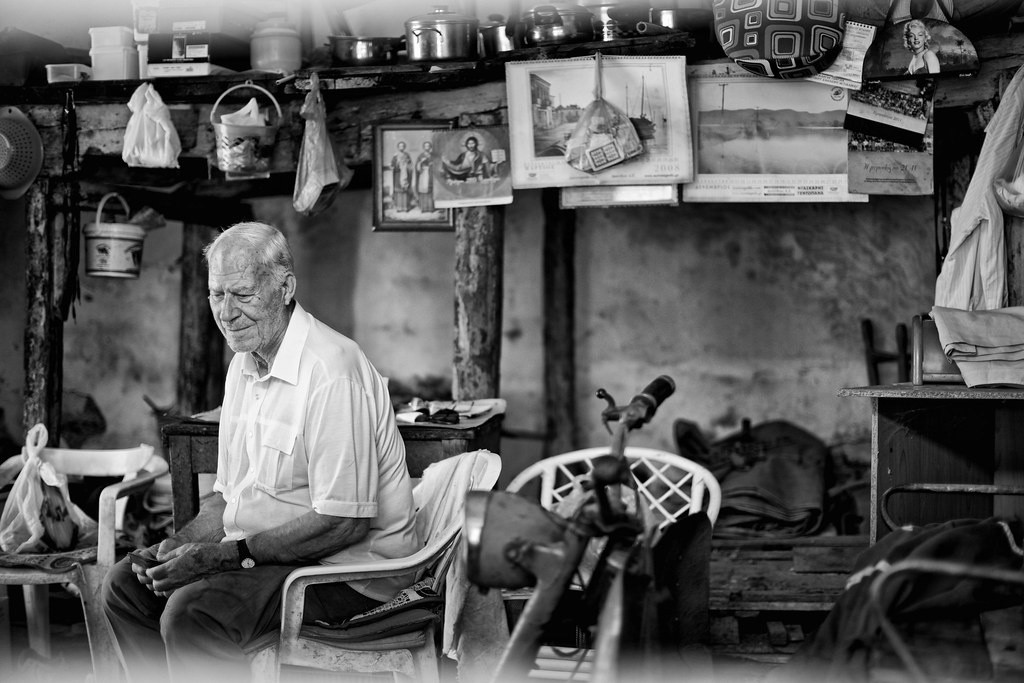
[369,117,458,235]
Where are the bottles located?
[251,19,302,74]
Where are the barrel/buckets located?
[211,85,279,177]
[80,192,144,278]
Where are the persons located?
[415,142,438,213]
[902,20,940,75]
[442,136,494,182]
[391,142,413,212]
[101,222,420,683]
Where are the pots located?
[480,0,713,58]
[324,34,405,66]
[405,4,480,63]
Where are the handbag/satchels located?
[122,84,181,168]
[293,71,355,219]
[565,54,643,171]
[0,423,99,555]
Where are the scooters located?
[459,376,1024,683]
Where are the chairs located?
[504,444,724,683]
[246,444,503,683]
[0,445,171,683]
[859,474,1024,683]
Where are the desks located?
[833,380,1024,548]
[157,401,508,534]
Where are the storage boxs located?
[43,0,267,84]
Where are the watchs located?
[237,539,257,569]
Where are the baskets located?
[1,107,43,198]
[209,85,282,175]
